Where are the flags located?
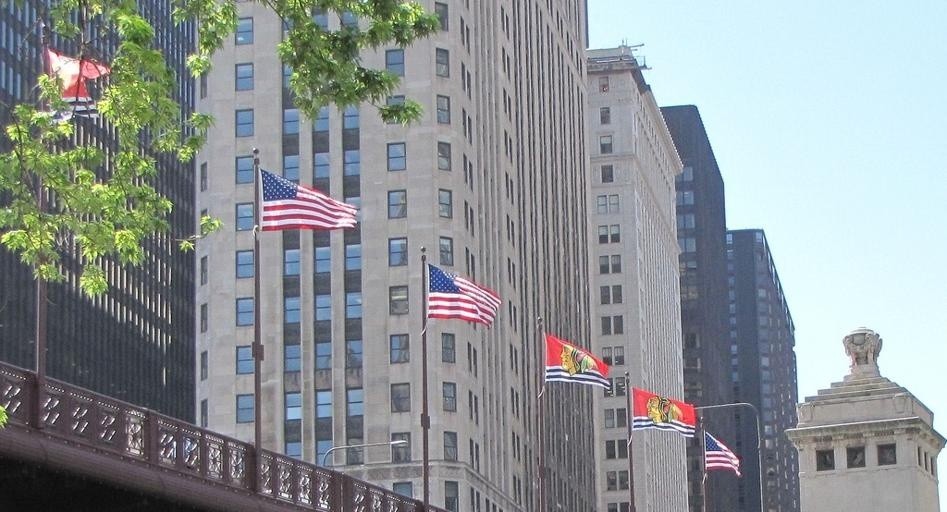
[428,263,501,328]
[545,331,609,392]
[48,48,112,119]
[258,169,357,231]
[705,432,740,477]
[630,386,696,438]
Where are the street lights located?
[320,439,407,469]
